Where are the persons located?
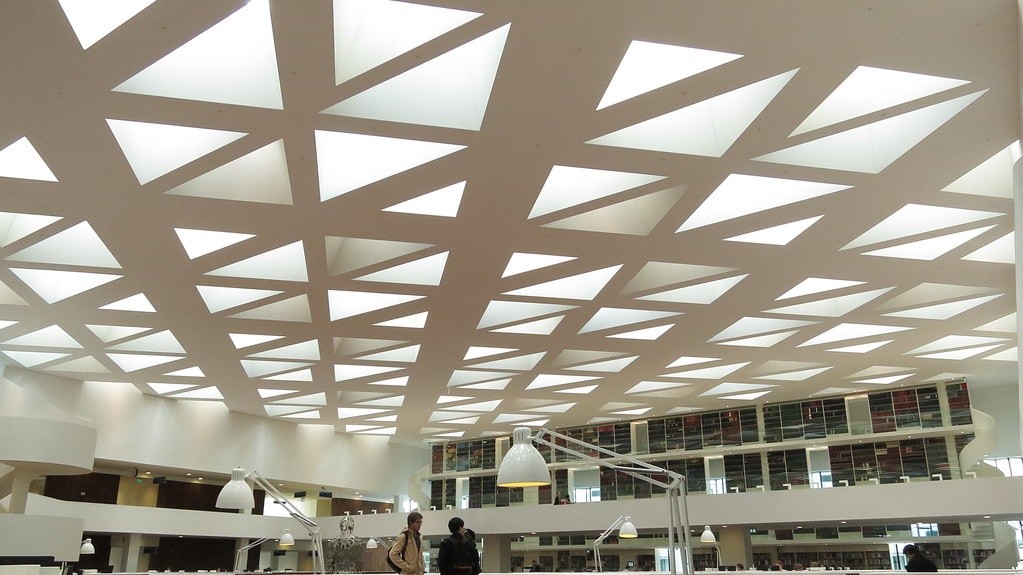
[794,564,804,570]
[555,490,561,505]
[566,495,571,504]
[903,544,938,573]
[388,511,427,575]
[775,560,788,572]
[437,517,482,575]
[530,560,541,572]
[736,563,745,571]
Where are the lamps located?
[496,426,695,575]
[592,516,639,573]
[366,535,390,551]
[61,537,95,572]
[232,525,294,572]
[700,526,722,571]
[215,466,327,575]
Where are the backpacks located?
[386,531,408,574]
[444,537,474,575]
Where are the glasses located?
[415,521,422,523]
